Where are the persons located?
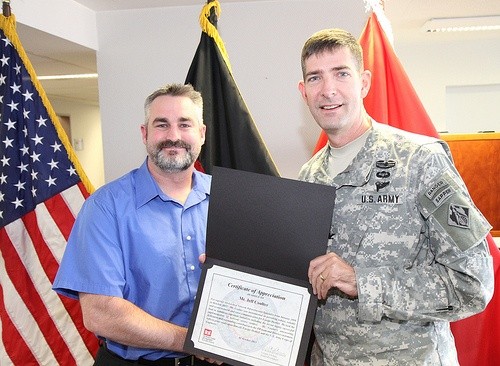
[198,26,496,366]
[50,83,234,366]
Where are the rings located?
[320,274,325,281]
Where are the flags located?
[312,0,500,365]
[180,0,281,177]
[0,0,107,366]
